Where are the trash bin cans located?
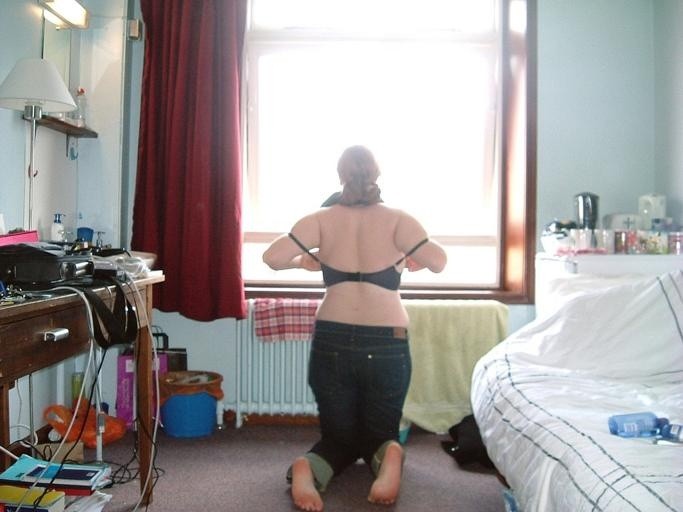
[161,370,224,439]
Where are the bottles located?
[96,232,102,248]
[610,413,683,443]
[51,214,64,242]
[74,88,87,128]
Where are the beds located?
[472,269,683,512]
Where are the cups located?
[572,229,615,254]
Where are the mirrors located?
[43,18,71,117]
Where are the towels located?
[397,300,510,436]
[255,298,320,343]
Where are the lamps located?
[29,0,88,30]
[0,58,77,232]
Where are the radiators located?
[216,299,323,429]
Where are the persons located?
[261,144,448,511]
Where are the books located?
[0,452,110,511]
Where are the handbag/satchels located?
[6,251,138,348]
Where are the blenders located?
[575,193,599,247]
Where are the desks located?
[534,252,683,319]
[0,274,166,512]
